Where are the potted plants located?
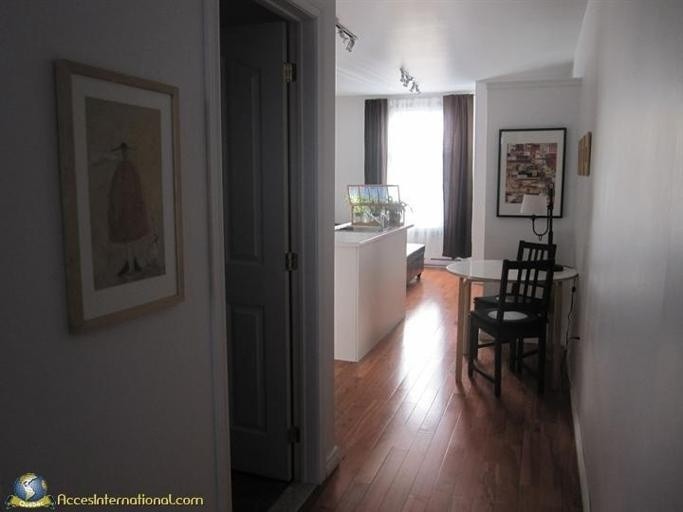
[350,197,414,226]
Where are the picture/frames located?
[497,128,566,218]
[56,59,185,335]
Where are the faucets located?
[364,209,388,231]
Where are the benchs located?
[407,242,425,282]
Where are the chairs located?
[468,240,557,399]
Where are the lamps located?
[400,66,421,95]
[335,16,358,52]
[520,183,563,271]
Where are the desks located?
[334,222,414,362]
[446,259,579,392]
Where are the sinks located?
[338,224,379,233]
